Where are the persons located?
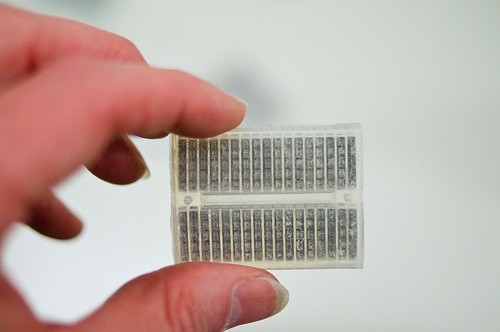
[0,0,289,332]
